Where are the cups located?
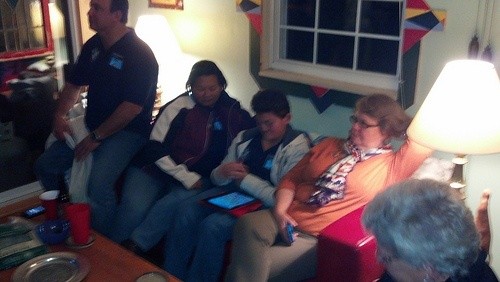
[39,190,60,222]
[37,220,70,245]
[66,203,90,245]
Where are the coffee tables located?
[0,195,181,282]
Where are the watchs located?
[91,130,104,143]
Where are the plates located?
[66,233,97,249]
[10,251,90,282]
[134,272,167,282]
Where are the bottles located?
[475,193,490,265]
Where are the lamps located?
[405,58,500,202]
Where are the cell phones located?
[23,204,48,218]
[287,223,293,242]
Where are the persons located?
[225,94,435,282]
[35,0,159,238]
[164,89,309,282]
[111,60,250,252]
[361,177,500,282]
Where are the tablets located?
[206,191,256,211]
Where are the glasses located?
[350,115,381,128]
[376,245,400,265]
